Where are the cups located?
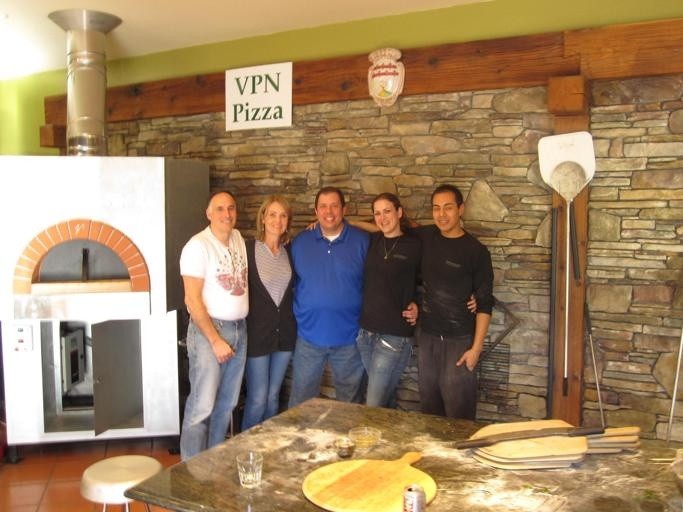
[333,438,356,457]
[235,452,263,488]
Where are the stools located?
[80,455,162,512]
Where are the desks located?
[124,397,683,512]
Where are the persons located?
[240,194,299,431]
[305,180,494,426]
[356,192,479,411]
[286,186,419,409]
[178,191,289,461]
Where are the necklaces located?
[381,236,399,260]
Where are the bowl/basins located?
[348,426,382,446]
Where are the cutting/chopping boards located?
[301,452,437,511]
[468,416,642,470]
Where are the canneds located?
[402,484,426,512]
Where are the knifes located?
[452,423,604,449]
